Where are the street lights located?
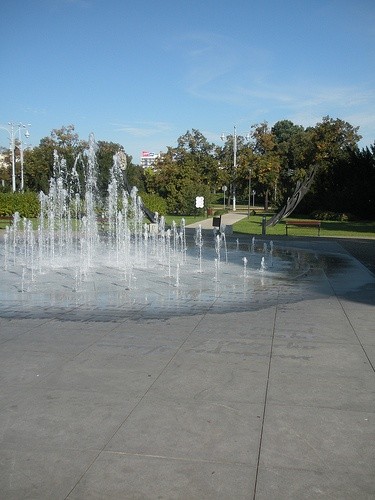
[221,125,250,212]
[1,121,31,193]
